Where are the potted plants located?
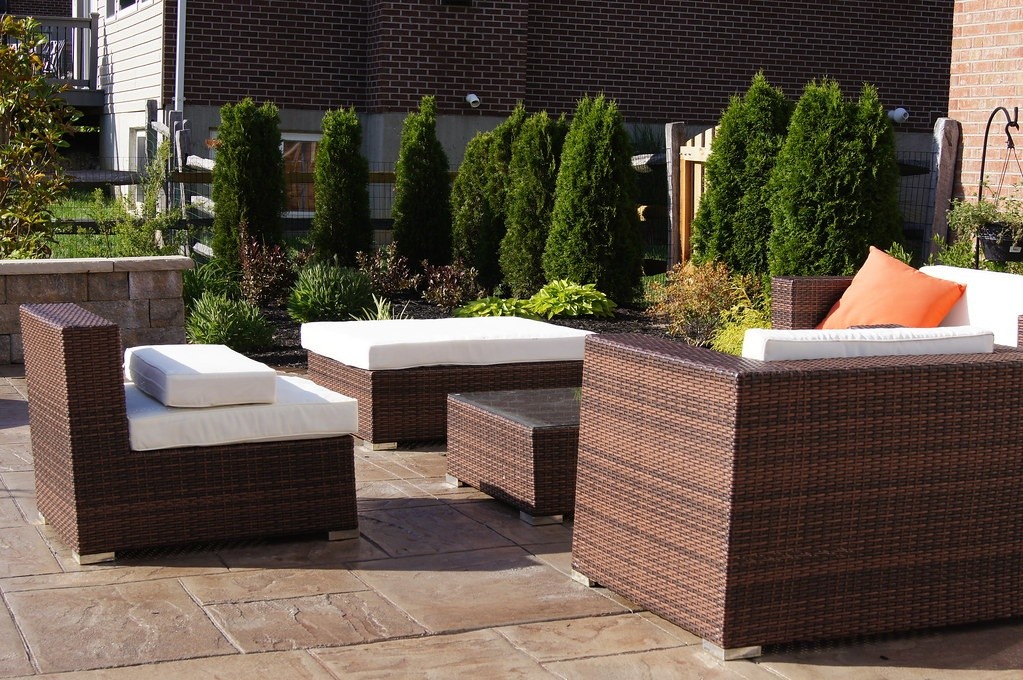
[945,174,1023,266]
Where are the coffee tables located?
[444,387,586,528]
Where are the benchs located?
[300,314,600,451]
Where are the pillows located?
[124,343,278,409]
[808,244,967,329]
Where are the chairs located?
[569,321,1023,663]
[9,38,68,78]
[19,301,363,567]
[766,265,1023,353]
